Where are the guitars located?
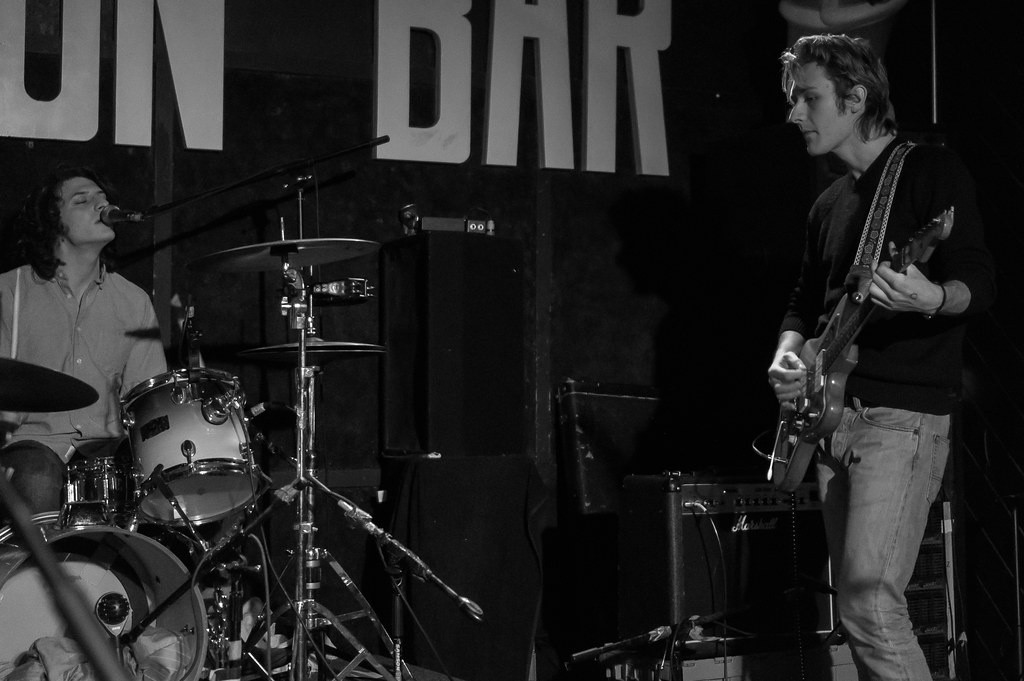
[774,205,956,494]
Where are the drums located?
[0,511,209,681]
[64,457,138,526]
[119,367,263,525]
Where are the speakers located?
[379,229,537,681]
[619,474,834,645]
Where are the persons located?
[767,35,995,681]
[0,164,168,517]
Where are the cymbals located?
[0,358,100,412]
[184,236,382,271]
[237,337,388,365]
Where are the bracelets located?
[922,281,946,320]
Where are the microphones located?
[244,401,271,421]
[100,205,144,224]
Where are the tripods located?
[126,135,484,681]
